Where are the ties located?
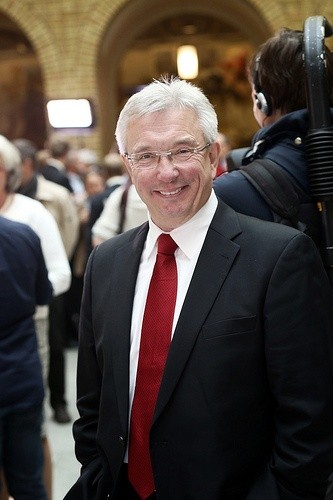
[126,232,181,500]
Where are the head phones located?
[252,52,273,118]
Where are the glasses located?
[123,142,212,170]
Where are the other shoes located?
[54,407,70,423]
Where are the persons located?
[213,27,333,278]
[0,132,55,500]
[63,73,333,500]
[0,134,228,425]
[0,194,70,500]
